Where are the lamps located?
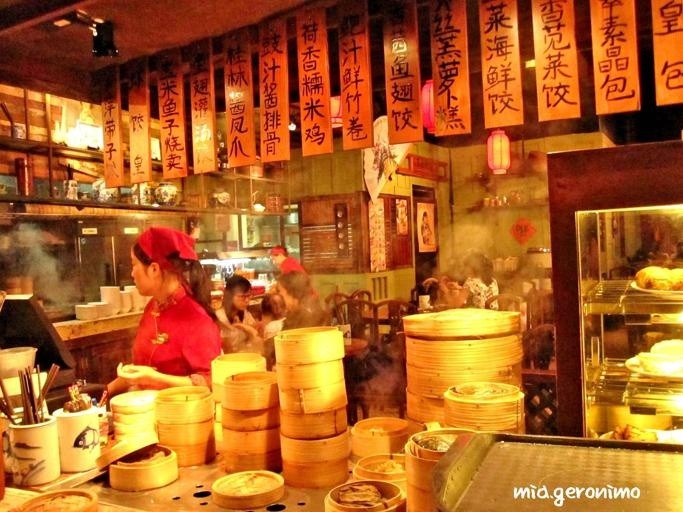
[488,126,526,176]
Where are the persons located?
[458,253,501,308]
[214,246,327,370]
[100,225,222,405]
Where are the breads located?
[636,266,683,291]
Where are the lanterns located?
[487,130,510,174]
[421,80,435,132]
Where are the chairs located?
[325,274,553,435]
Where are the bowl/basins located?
[101,285,148,315]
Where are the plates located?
[599,428,670,441]
[624,355,683,381]
[630,280,683,300]
[75,301,110,322]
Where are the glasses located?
[234,293,253,301]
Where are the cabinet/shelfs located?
[465,169,547,220]
[1,79,292,217]
[543,137,683,444]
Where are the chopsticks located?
[1,362,60,424]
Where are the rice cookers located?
[524,246,552,269]
